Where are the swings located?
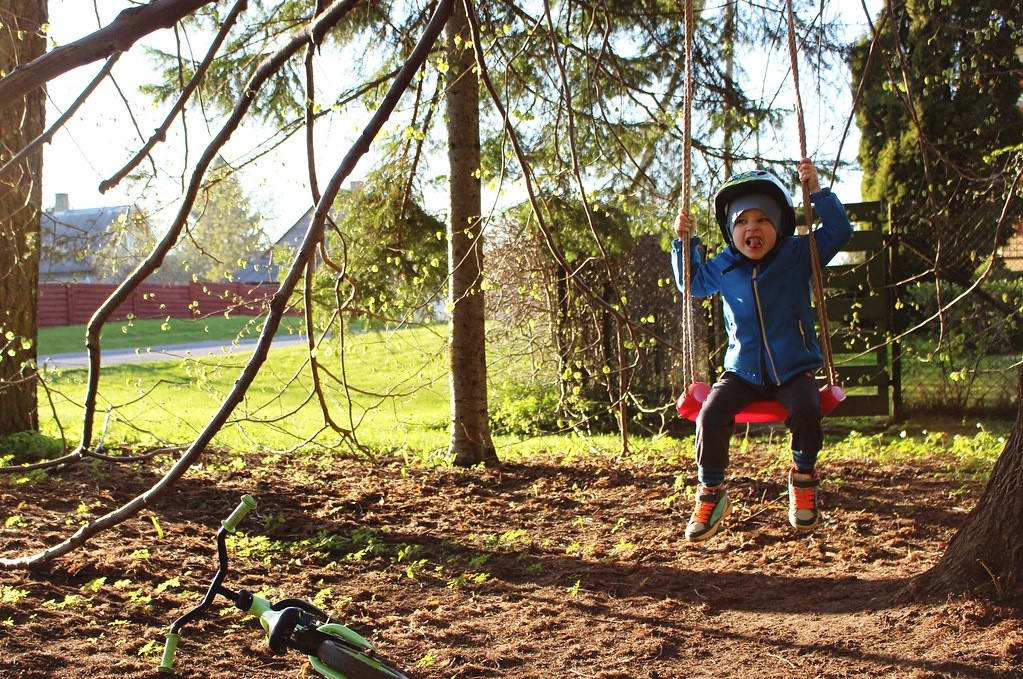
[673,1,850,425]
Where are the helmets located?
[714,171,795,244]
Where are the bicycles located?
[153,495,403,678]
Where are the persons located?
[670,157,854,543]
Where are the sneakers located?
[684,483,733,543]
[787,467,820,530]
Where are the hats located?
[727,193,781,239]
